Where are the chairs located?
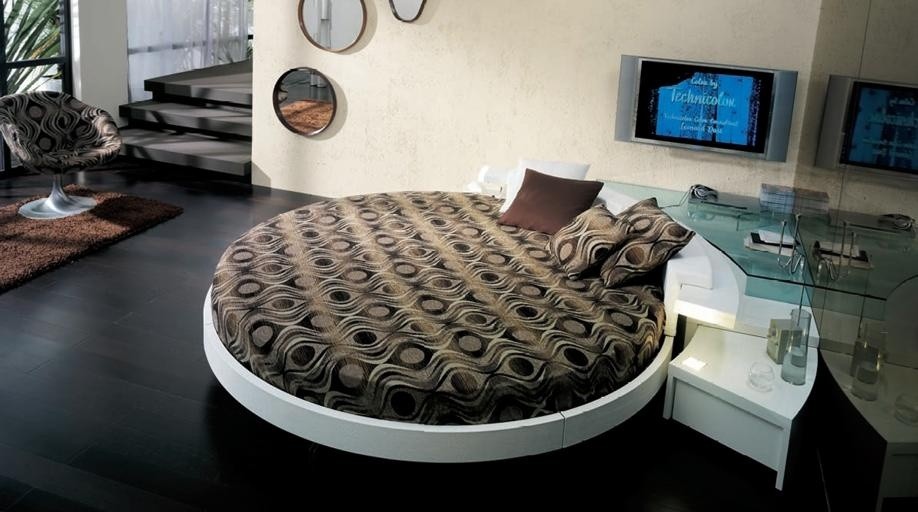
[0,90,123,220]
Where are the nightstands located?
[800,209,911,512]
[600,179,819,491]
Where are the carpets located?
[0,183,185,295]
[281,100,332,131]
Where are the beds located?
[202,190,676,463]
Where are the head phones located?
[690,182,719,202]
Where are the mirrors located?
[298,0,367,52]
[792,2,918,510]
[272,67,337,138]
[388,0,426,22]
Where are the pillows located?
[599,197,696,289]
[544,203,636,281]
[496,168,604,236]
[498,159,592,214]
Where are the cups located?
[747,362,777,394]
[893,393,918,426]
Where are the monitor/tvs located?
[613,55,799,162]
[814,74,917,179]
